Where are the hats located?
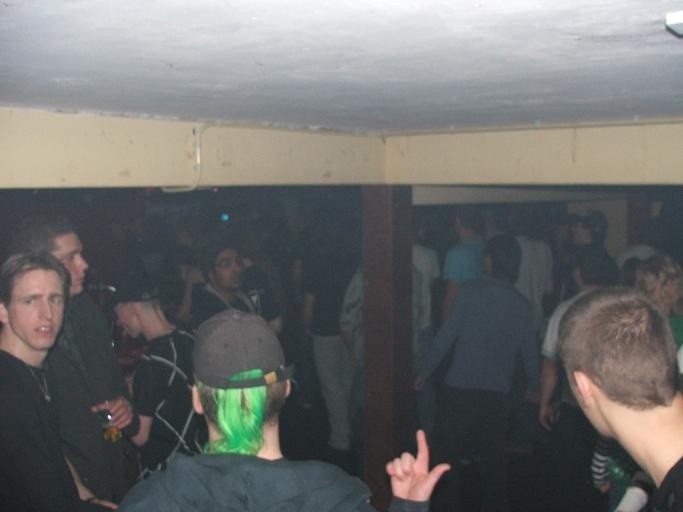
[191,308,288,389]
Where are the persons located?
[64,187,682,512]
[7,210,154,511]
[0,249,121,512]
